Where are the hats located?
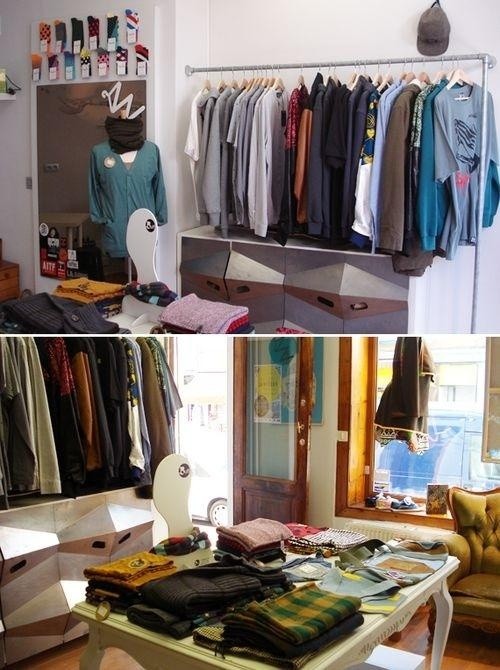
[417,1,450,56]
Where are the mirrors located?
[31,81,146,293]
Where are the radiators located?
[343,518,454,545]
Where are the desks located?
[40,211,90,250]
[71,520,460,668]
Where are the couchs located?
[428,487,499,646]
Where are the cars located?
[188,464,229,527]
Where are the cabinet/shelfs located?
[176,225,426,335]
[0,474,154,670]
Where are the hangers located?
[200,54,480,104]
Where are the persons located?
[88,110,168,259]
[372,337,436,455]
